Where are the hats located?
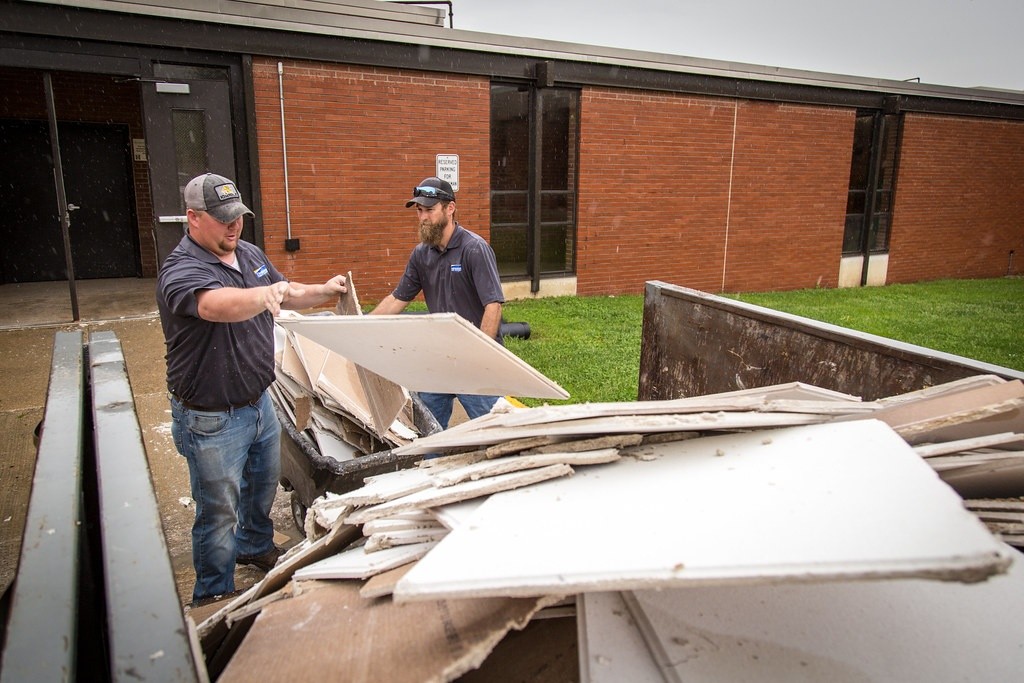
[184,173,255,224]
[405,177,455,208]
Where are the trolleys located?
[272,387,445,538]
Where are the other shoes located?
[235,546,287,572]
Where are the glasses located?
[414,186,455,199]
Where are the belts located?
[172,393,263,412]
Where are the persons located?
[156,172,348,601]
[364,177,505,460]
